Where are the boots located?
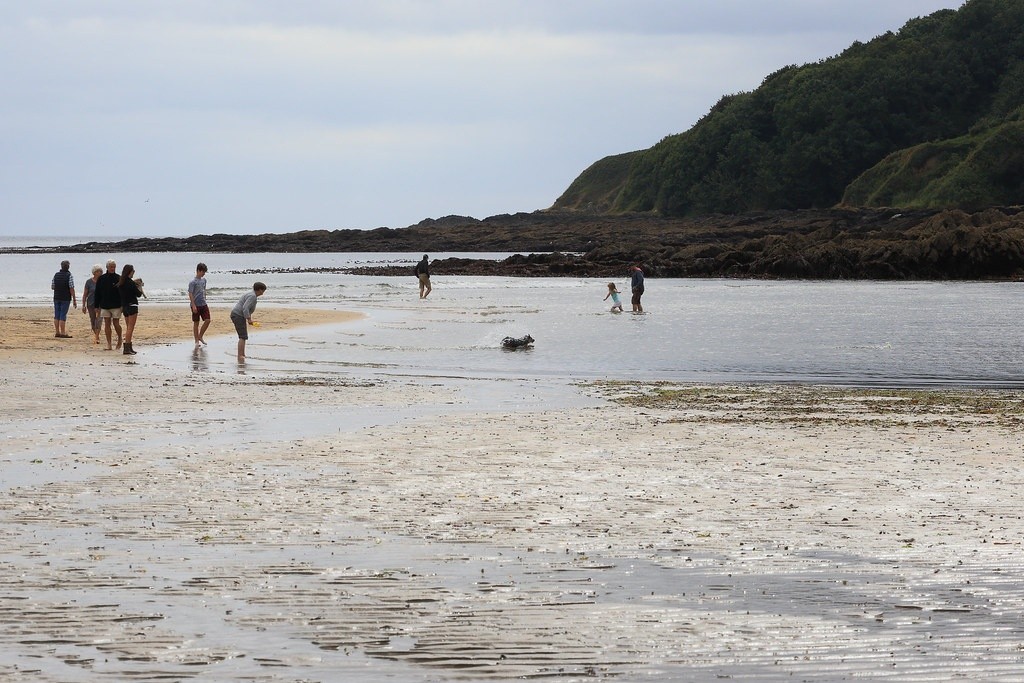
[123,342,137,355]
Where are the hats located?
[627,262,635,266]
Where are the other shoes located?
[60,334,72,338]
[55,333,61,336]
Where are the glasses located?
[130,270,135,274]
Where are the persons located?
[415,254,431,299]
[119,265,142,355]
[95,260,123,350]
[188,263,211,345]
[230,282,266,356]
[603,283,624,312]
[51,261,77,338]
[629,260,645,311]
[82,266,103,343]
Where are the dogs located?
[500,334,535,348]
